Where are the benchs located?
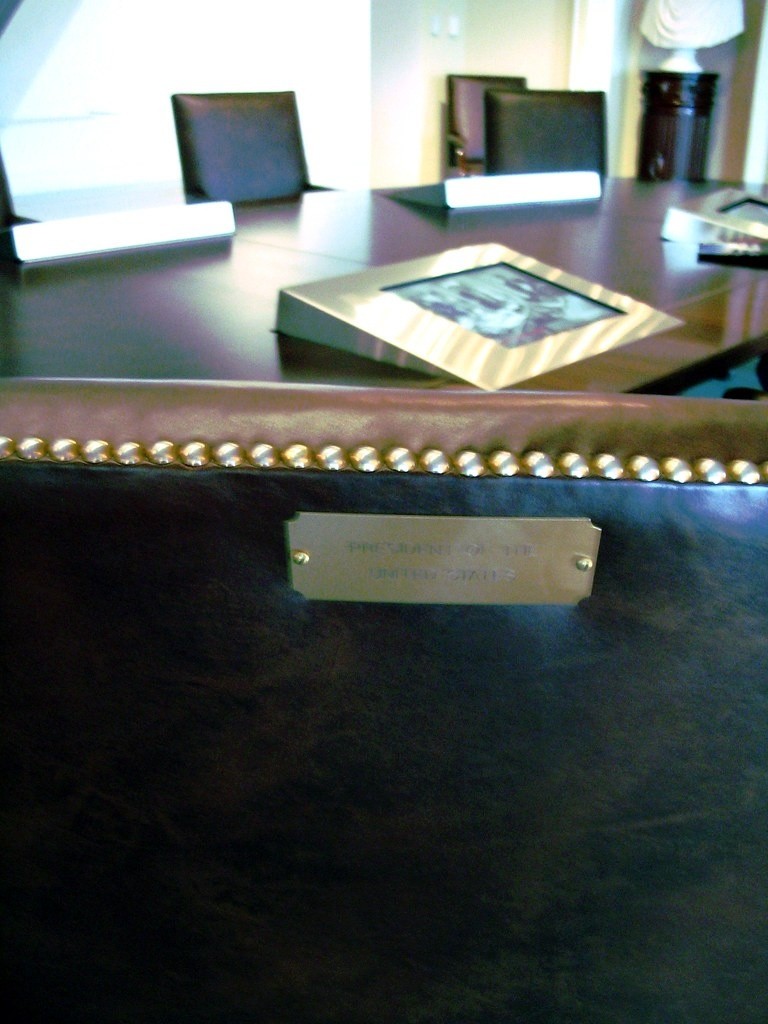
[0,371,768,1024]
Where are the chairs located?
[171,90,333,210]
[484,88,608,181]
[439,75,529,180]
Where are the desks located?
[0,184,768,396]
